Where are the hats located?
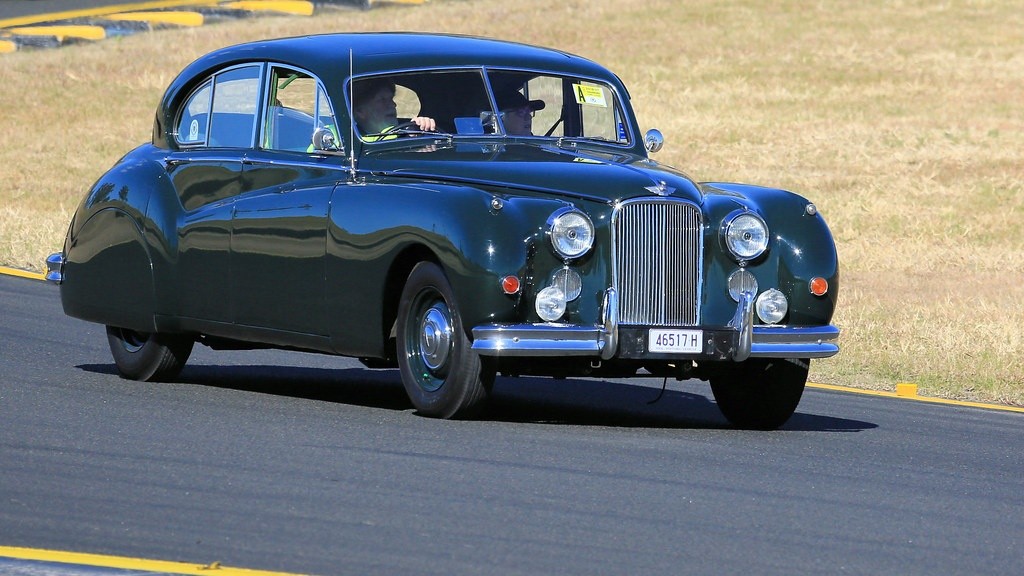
[484,87,546,111]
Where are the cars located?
[41,31,844,428]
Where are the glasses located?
[505,108,536,118]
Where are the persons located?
[306,78,441,154]
[486,90,544,137]
